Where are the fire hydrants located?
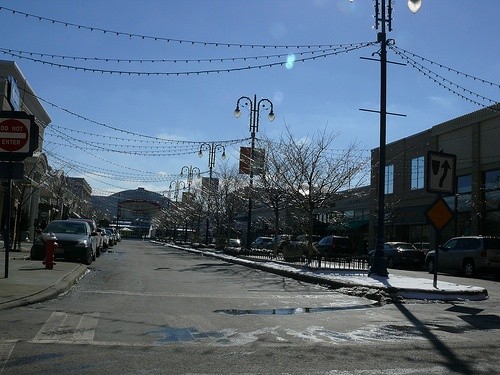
[42,231,58,269]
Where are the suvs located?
[425,235,500,278]
[316,234,353,261]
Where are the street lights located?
[344,0,422,278]
[179,164,201,246]
[162,178,186,246]
[233,94,275,256]
[198,142,226,246]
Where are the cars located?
[366,241,426,270]
[30,217,122,265]
[250,234,321,253]
[222,238,241,253]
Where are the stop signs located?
[0,119,29,151]
